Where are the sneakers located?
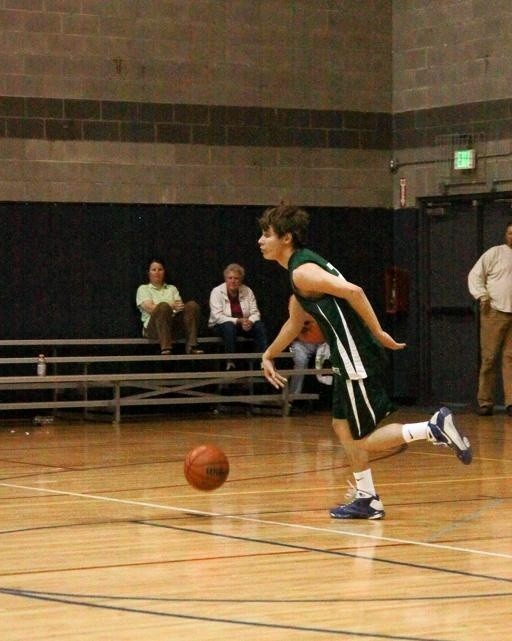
[226,360,236,371]
[161,350,172,354]
[476,405,512,416]
[191,349,204,354]
[329,480,385,519]
[427,407,472,464]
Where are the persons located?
[256,197,473,520]
[135,255,207,355]
[286,295,327,415]
[204,261,269,371]
[466,220,512,417]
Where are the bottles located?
[37,354,47,376]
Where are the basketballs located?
[184,446,229,491]
